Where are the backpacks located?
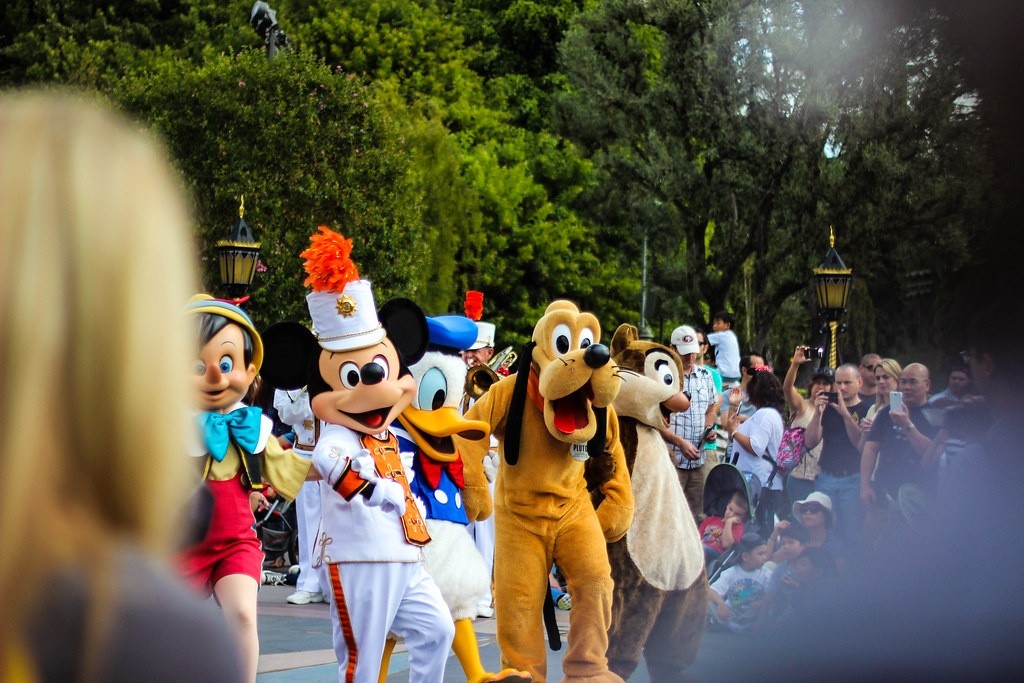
[766,418,805,472]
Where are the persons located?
[547,562,571,610]
[663,312,1024,683]
[243,375,332,604]
[454,291,517,619]
[168,293,324,683]
[0,85,248,683]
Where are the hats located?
[812,367,834,380]
[959,346,977,354]
[792,492,832,528]
[467,322,496,350]
[671,326,700,355]
[730,532,767,561]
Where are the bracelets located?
[904,423,914,432]
[768,536,776,543]
[730,430,737,440]
[730,407,738,411]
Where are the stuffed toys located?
[258,226,710,683]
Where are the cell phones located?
[821,392,838,402]
[804,349,822,358]
[890,392,902,411]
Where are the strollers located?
[690,452,778,582]
[256,492,299,565]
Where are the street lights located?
[814,226,851,369]
[212,194,264,306]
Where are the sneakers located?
[261,571,286,586]
[287,591,323,604]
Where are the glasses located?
[863,365,874,371]
[699,341,705,346]
[800,506,821,513]
